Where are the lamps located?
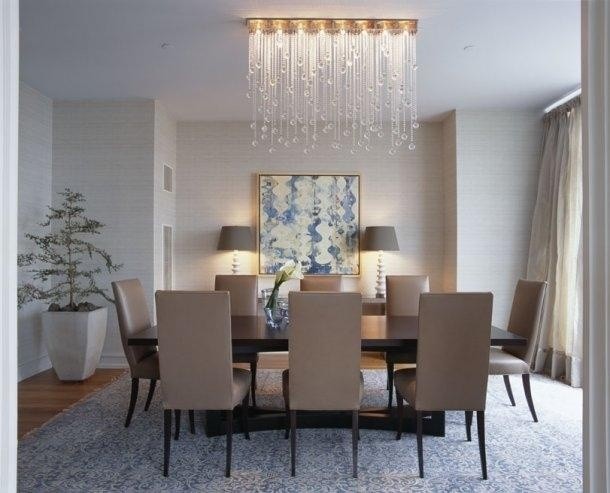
[216,223,260,280]
[358,225,405,299]
[244,13,420,162]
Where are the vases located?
[259,288,296,331]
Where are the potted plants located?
[20,187,121,382]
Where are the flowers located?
[265,257,302,313]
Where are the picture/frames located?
[257,171,363,278]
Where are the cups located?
[263,305,287,329]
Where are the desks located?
[124,308,535,442]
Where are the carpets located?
[19,365,591,493]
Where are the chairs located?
[298,273,343,296]
[213,272,262,391]
[154,287,253,481]
[480,278,550,425]
[279,290,366,483]
[383,273,433,408]
[109,275,201,439]
[387,289,495,485]
